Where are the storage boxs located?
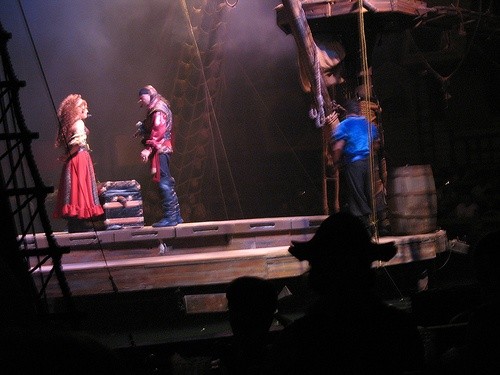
[81,180,145,230]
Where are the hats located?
[289,212,397,263]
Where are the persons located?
[0,329,125,375]
[273,212,500,375]
[224,277,278,375]
[330,100,380,217]
[133,84,184,228]
[52,93,105,234]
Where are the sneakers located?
[152,217,184,227]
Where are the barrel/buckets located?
[387,164,438,235]
